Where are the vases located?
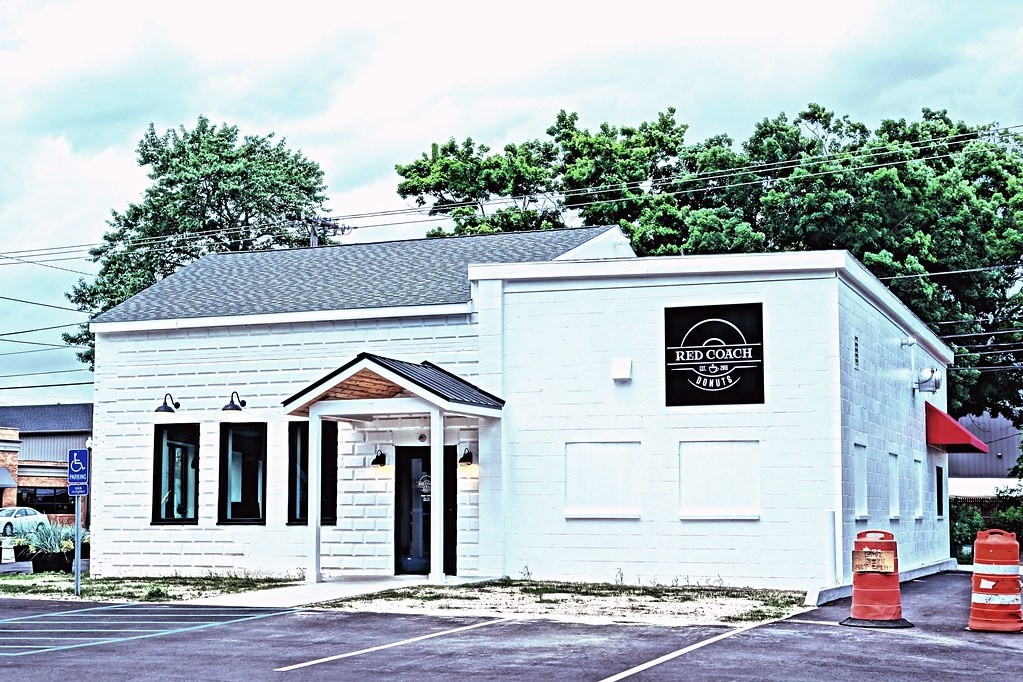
[66,543,90,559]
[31,553,73,574]
[14,545,32,562]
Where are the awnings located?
[0,467,17,487]
[927,400,989,456]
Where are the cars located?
[0,507,49,537]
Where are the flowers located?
[9,511,90,563]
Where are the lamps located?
[371,450,386,466]
[611,358,633,381]
[459,448,473,467]
[155,394,180,412]
[222,392,246,410]
